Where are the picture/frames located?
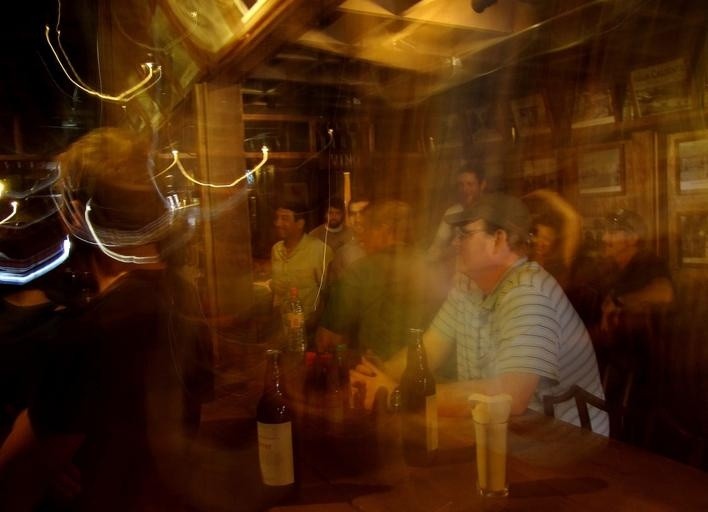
[414,51,708,271]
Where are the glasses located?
[457,228,488,238]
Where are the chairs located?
[542,351,640,446]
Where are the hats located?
[443,192,533,238]
[602,204,649,241]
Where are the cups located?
[470,413,510,494]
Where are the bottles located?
[331,344,357,420]
[255,347,299,500]
[402,326,438,467]
[279,285,308,356]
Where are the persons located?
[1,126,675,511]
